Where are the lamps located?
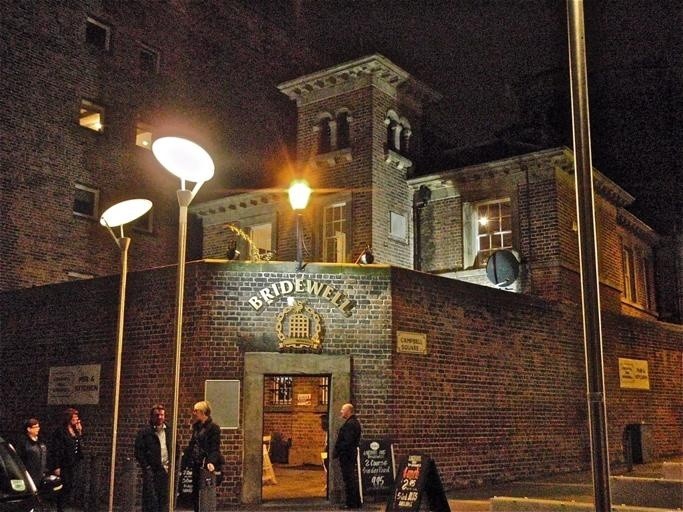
[289,179,310,272]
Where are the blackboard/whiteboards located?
[358,445,395,494]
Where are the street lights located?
[99,197,154,510]
[151,135,216,511]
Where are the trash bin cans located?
[629,422,654,464]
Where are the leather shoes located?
[340,503,361,510]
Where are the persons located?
[331,402,361,508]
[13,418,47,490]
[133,404,170,511]
[49,407,84,511]
[175,401,225,512]
